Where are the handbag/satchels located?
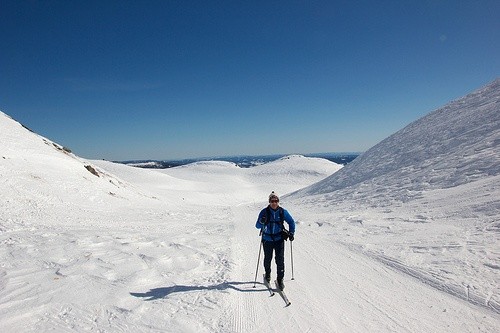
[281,229,289,240]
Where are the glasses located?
[270,200,278,203]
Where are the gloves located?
[261,215,266,223]
[288,232,294,241]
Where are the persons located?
[256,191,295,290]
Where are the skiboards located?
[263,274,291,306]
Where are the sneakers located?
[266,274,270,283]
[277,277,285,290]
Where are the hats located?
[269,191,279,203]
